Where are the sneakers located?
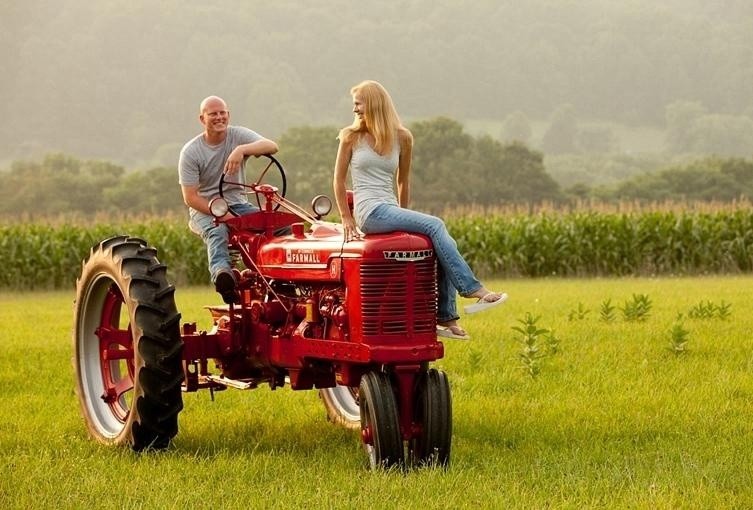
[215,269,238,303]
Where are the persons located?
[332,81,507,342]
[176,96,283,302]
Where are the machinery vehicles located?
[66,147,456,486]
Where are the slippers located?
[437,326,470,340]
[464,291,507,314]
